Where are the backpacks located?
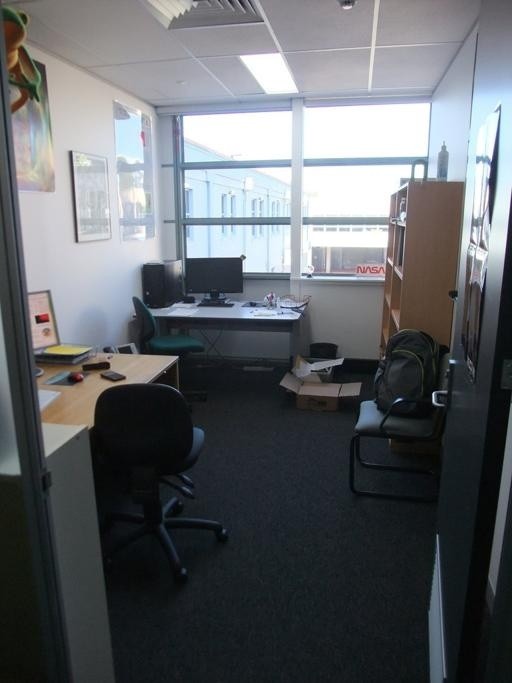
[374,328,441,417]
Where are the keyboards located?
[38,388,63,411]
[197,302,234,307]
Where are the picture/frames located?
[26,290,60,351]
[71,150,112,243]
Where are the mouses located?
[67,372,84,382]
[249,301,256,306]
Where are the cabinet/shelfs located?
[379,181,464,355]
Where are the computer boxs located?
[142,258,184,308]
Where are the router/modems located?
[183,293,195,303]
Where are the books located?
[32,345,92,365]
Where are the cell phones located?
[101,369,126,381]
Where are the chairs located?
[94,383,226,583]
[348,353,452,504]
[132,296,208,412]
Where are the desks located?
[34,354,179,432]
[133,291,311,365]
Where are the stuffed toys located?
[0,4,43,117]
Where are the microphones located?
[83,361,111,371]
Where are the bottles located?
[437,140,449,181]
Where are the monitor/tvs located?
[27,289,62,377]
[184,257,244,302]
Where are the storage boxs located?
[278,371,362,411]
[292,358,334,382]
